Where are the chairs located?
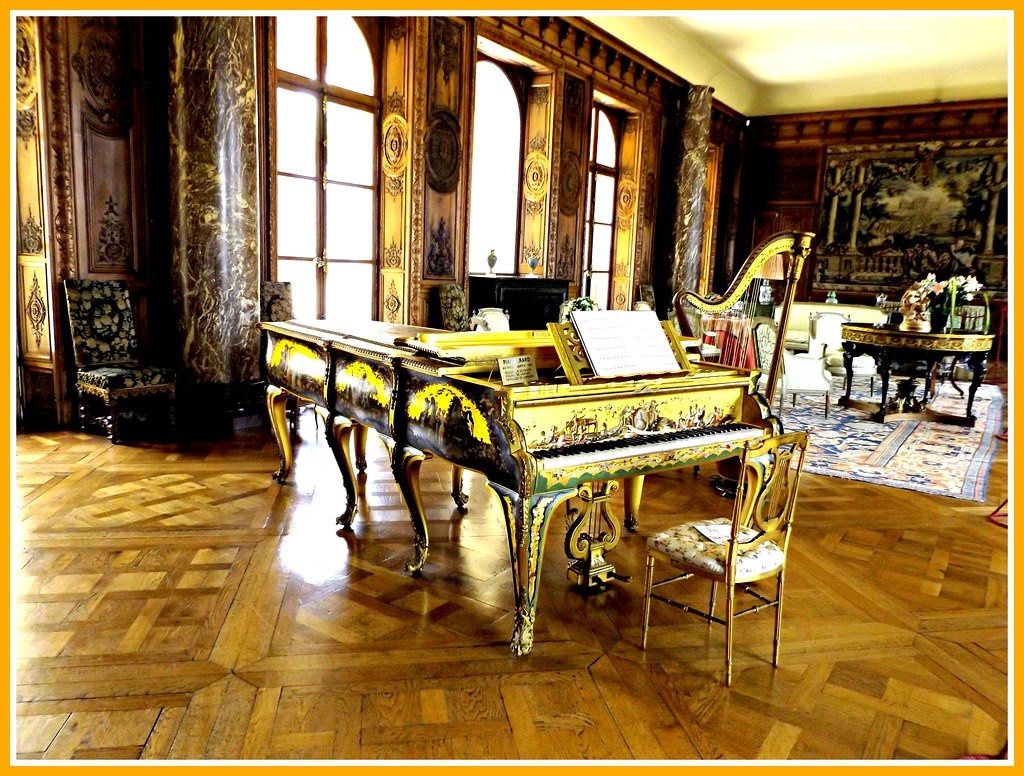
[752,323,830,420]
[64,277,174,445]
[666,307,721,363]
[262,281,315,435]
[808,311,876,398]
[641,430,812,689]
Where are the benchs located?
[770,301,883,357]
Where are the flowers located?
[523,239,543,263]
[558,297,604,323]
[931,273,983,331]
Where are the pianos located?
[253,315,784,657]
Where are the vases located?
[932,309,950,333]
[527,257,538,275]
[487,250,497,270]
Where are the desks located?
[838,322,995,428]
